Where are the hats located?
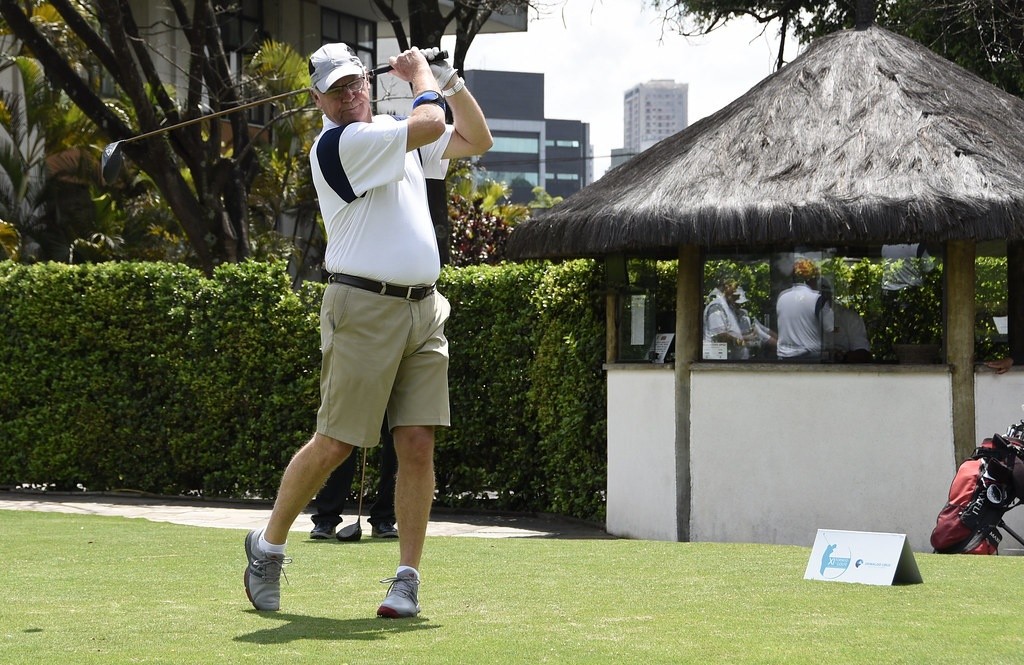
[733,287,748,303]
[309,43,362,93]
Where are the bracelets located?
[411,90,446,112]
[442,78,466,96]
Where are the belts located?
[334,273,436,301]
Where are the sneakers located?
[310,523,336,539]
[377,568,420,618]
[244,528,292,611]
[372,523,398,538]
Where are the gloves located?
[420,47,458,91]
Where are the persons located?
[701,240,934,364]
[244,42,495,616]
[308,411,398,538]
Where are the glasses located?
[326,76,364,98]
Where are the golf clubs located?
[101,48,452,181]
[334,446,368,543]
